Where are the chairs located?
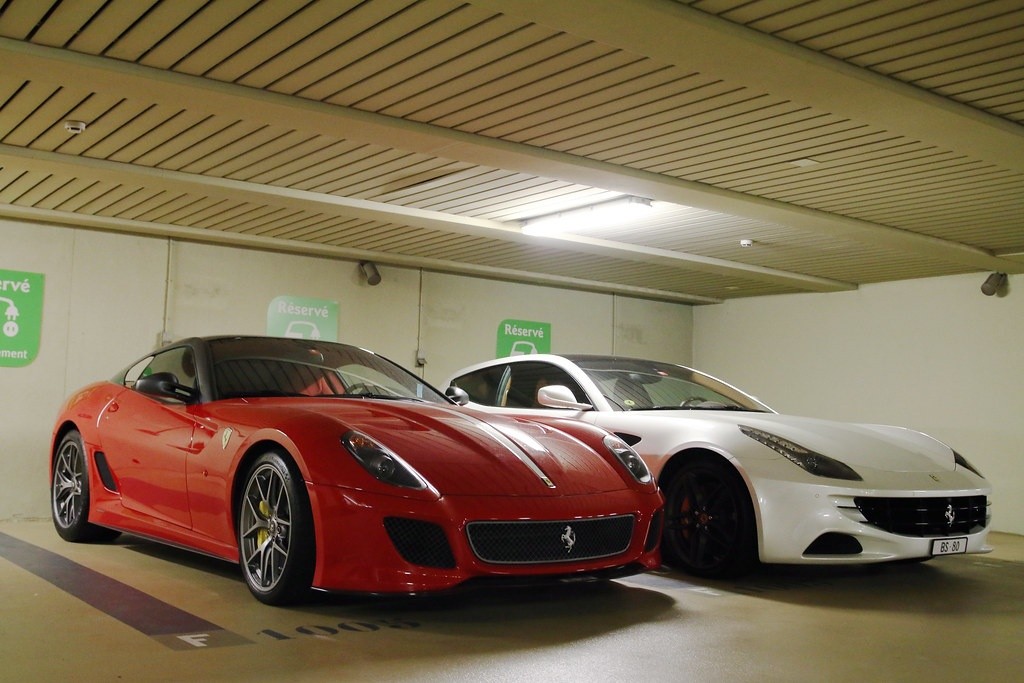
[613,378,654,411]
[282,348,331,395]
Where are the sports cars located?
[436,352,996,582]
[48,332,666,606]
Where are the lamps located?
[520,195,654,236]
